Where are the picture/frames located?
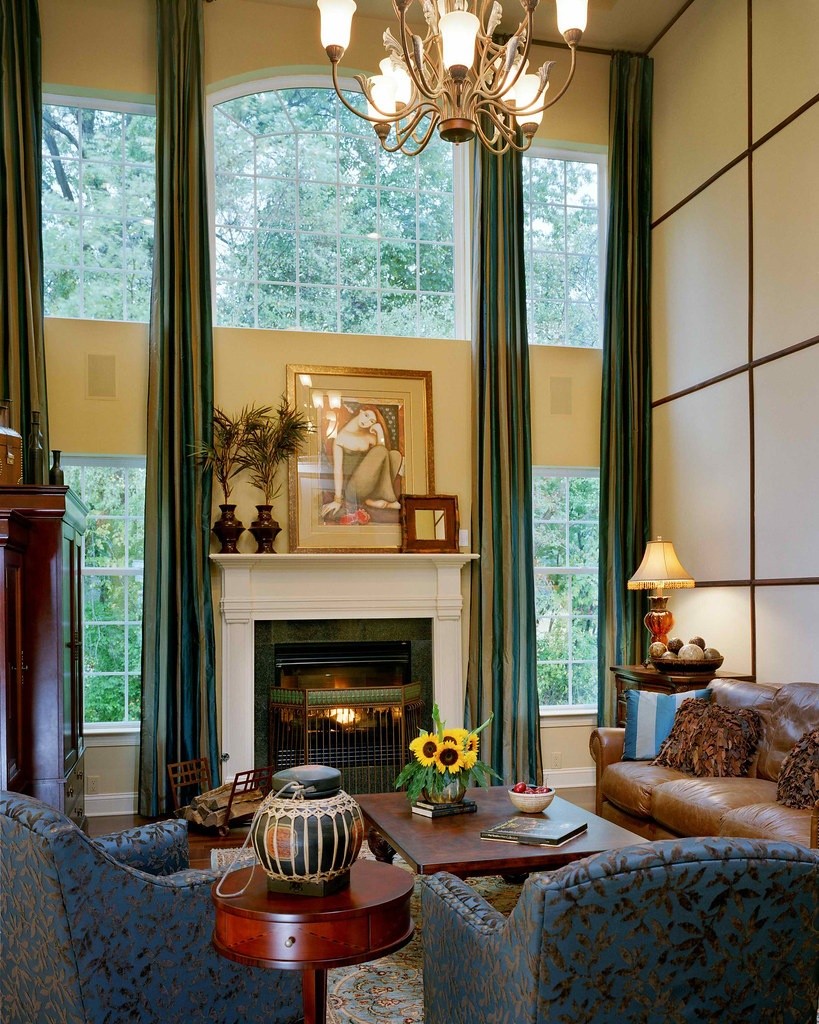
[287,364,463,556]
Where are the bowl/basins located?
[508,785,555,813]
[650,657,724,675]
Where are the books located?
[412,799,477,818]
[480,816,587,844]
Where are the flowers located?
[393,704,504,806]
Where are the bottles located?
[49,450,63,485]
[28,410,44,485]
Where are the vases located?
[421,775,468,804]
[214,504,282,554]
[27,411,64,486]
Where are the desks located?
[210,858,415,1024]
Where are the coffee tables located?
[351,785,652,879]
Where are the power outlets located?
[87,776,99,794]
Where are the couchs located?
[1,676,819,1024]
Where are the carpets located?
[210,837,523,1024]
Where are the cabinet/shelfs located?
[609,665,756,729]
[2,485,90,832]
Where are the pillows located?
[620,687,712,760]
[776,726,819,811]
[645,697,764,777]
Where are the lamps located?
[315,0,589,154]
[627,537,695,668]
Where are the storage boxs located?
[0,426,24,487]
